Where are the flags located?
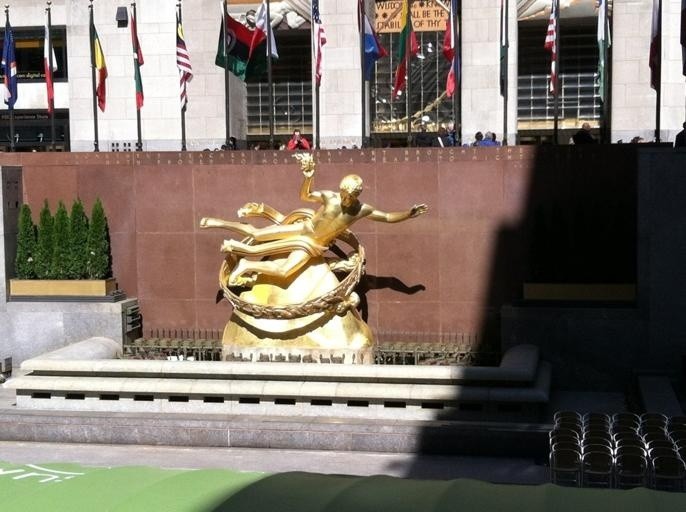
[89,10,108,112]
[355,1,388,81]
[594,1,614,103]
[254,1,280,58]
[41,12,60,117]
[545,2,566,96]
[391,2,425,95]
[174,5,192,116]
[309,1,327,88]
[130,6,148,109]
[213,10,265,82]
[0,16,18,112]
[442,0,467,96]
[649,0,663,94]
[500,1,510,99]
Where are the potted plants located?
[7,196,119,297]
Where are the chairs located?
[547,409,686,492]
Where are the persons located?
[226,136,240,151]
[407,124,501,149]
[675,122,686,147]
[200,156,429,283]
[286,128,312,151]
[574,122,600,144]
